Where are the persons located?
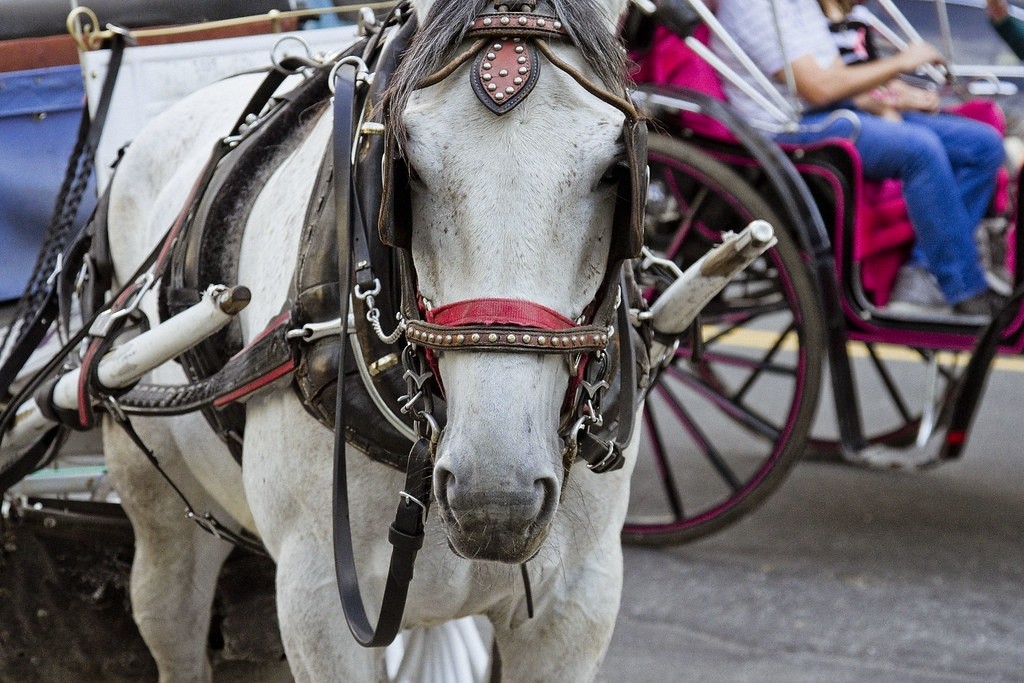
[794,0,1022,306]
[709,0,1016,323]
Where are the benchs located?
[609,0,1002,261]
[0,0,340,301]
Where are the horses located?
[88,0,645,683]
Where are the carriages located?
[1,0,783,683]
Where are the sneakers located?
[888,215,1014,323]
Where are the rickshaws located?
[620,0,1023,551]
